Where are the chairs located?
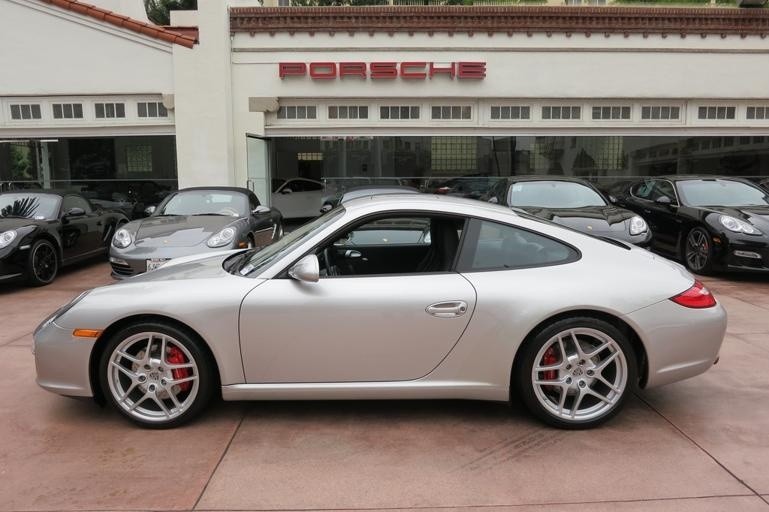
[419,219,458,272]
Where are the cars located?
[106,184,283,282]
[600,174,769,280]
[77,180,170,222]
[0,186,129,293]
[478,174,656,259]
[421,172,499,203]
[270,175,336,223]
[319,181,420,216]
[30,187,728,432]
